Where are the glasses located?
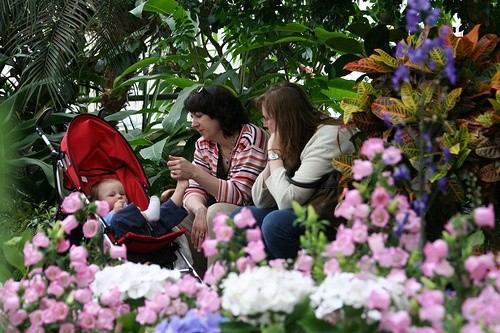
[197,86,207,96]
[260,117,276,123]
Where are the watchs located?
[267,151,283,161]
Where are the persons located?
[228,82,361,259]
[88,178,191,265]
[167,85,268,253]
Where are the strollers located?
[33,107,205,285]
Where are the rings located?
[198,233,205,238]
